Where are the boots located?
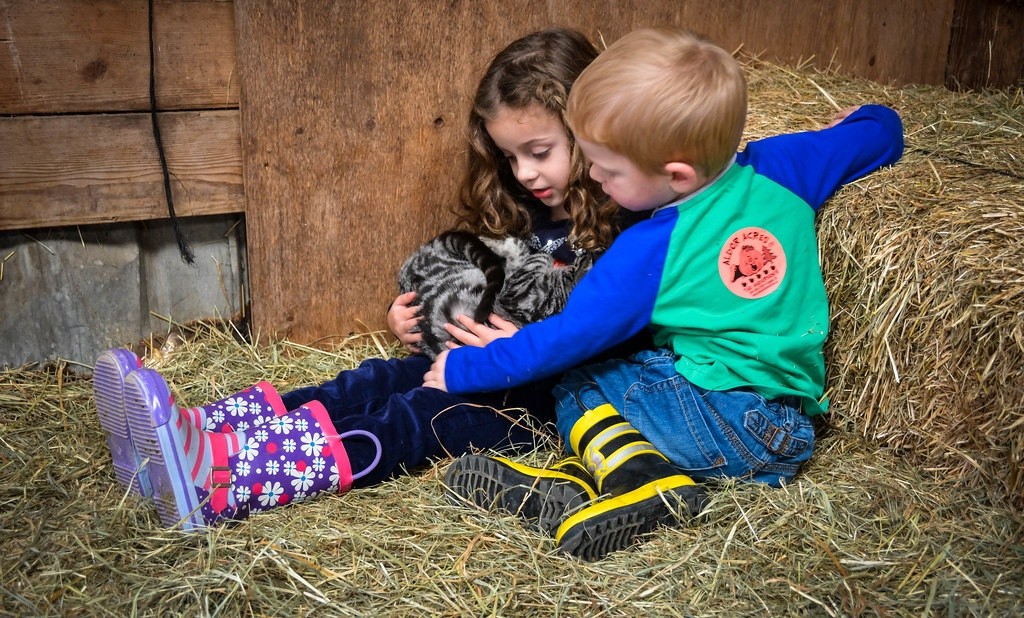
[93,348,288,497]
[123,368,382,535]
[443,453,602,540]
[556,382,713,561]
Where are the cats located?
[397,229,591,362]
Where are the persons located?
[421,27,905,563]
[93,29,654,533]
